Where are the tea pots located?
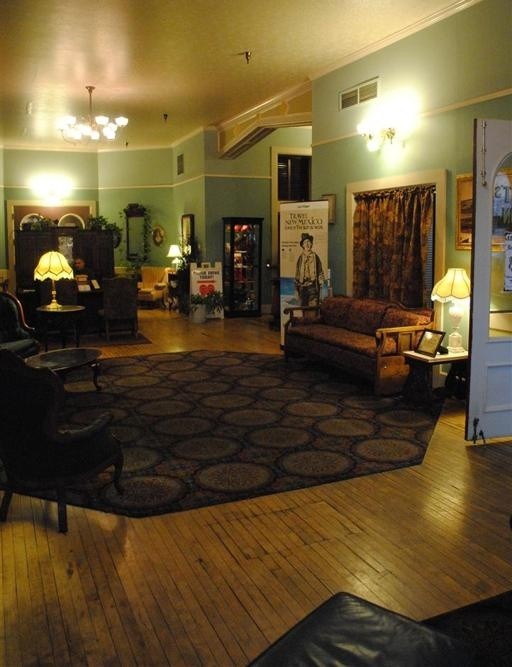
[123,202,150,262]
[182,213,194,258]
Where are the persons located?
[153,228,163,246]
[295,232,322,318]
[74,256,96,278]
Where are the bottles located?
[299,233,314,246]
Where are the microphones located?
[248,591,472,666]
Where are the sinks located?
[114,266,175,307]
[281,296,436,397]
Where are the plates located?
[79,229,115,334]
[14,226,81,336]
[222,216,264,318]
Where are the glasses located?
[55,85,129,143]
[356,115,395,153]
[33,249,74,308]
[428,267,470,352]
[166,245,182,270]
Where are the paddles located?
[1,349,445,520]
[38,329,154,349]
[417,589,512,667]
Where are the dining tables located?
[190,293,207,324]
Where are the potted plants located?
[455,167,511,251]
[414,327,448,357]
[320,192,337,224]
[6,199,98,269]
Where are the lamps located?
[1,348,125,535]
[1,291,41,359]
[97,277,138,342]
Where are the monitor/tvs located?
[74,274,91,292]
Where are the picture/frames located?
[36,304,86,351]
[166,272,188,312]
[401,346,469,418]
